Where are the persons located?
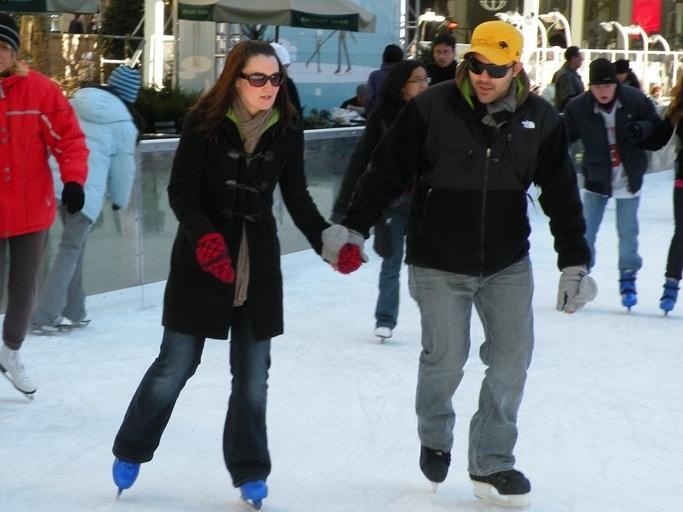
[341,20,600,508]
[29,66,142,333]
[0,13,90,394]
[69,14,84,34]
[111,39,362,500]
[321,20,683,343]
[269,42,306,124]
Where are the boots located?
[659,279,679,312]
[620,272,636,307]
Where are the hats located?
[108,65,142,104]
[383,44,403,65]
[461,20,524,67]
[588,57,619,85]
[0,12,21,53]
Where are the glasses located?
[467,57,516,78]
[236,70,286,88]
[408,76,432,85]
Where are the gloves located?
[556,266,598,315]
[321,224,368,274]
[540,83,555,106]
[62,181,86,215]
[330,210,348,224]
[624,122,641,145]
[195,232,236,285]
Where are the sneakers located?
[374,326,392,338]
[241,480,269,502]
[112,456,141,490]
[32,314,92,333]
[419,444,452,483]
[470,468,531,496]
[0,341,36,393]
